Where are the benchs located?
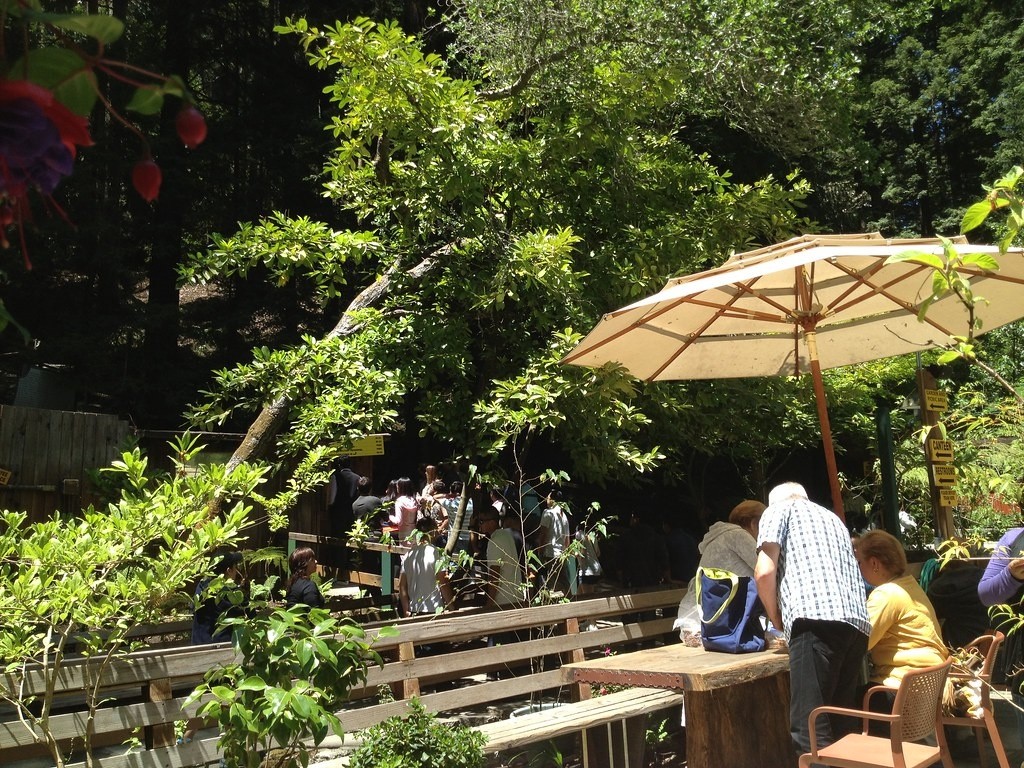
[0,586,684,768]
[905,559,1009,697]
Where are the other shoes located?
[579,621,598,631]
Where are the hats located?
[210,550,243,573]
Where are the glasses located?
[479,519,495,525]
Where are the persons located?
[754,481,870,768]
[848,529,948,740]
[849,532,875,590]
[328,455,602,701]
[977,491,1024,768]
[285,547,324,610]
[698,500,767,578]
[191,552,256,644]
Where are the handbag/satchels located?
[941,647,985,720]
[695,566,766,653]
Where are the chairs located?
[942,628,1011,768]
[798,656,954,768]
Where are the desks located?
[560,640,793,768]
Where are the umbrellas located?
[556,230,1024,525]
